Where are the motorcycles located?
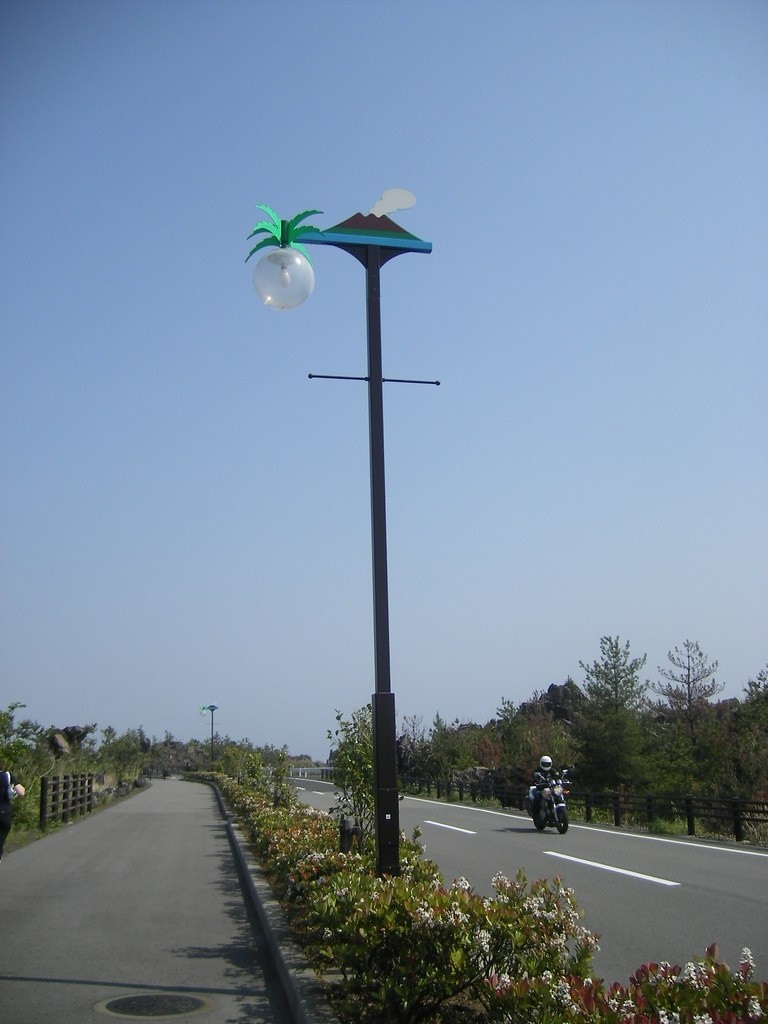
[525,770,572,834]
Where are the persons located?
[532,755,569,820]
[0,762,25,861]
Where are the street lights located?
[244,189,440,879]
[199,704,219,772]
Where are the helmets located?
[540,756,552,771]
